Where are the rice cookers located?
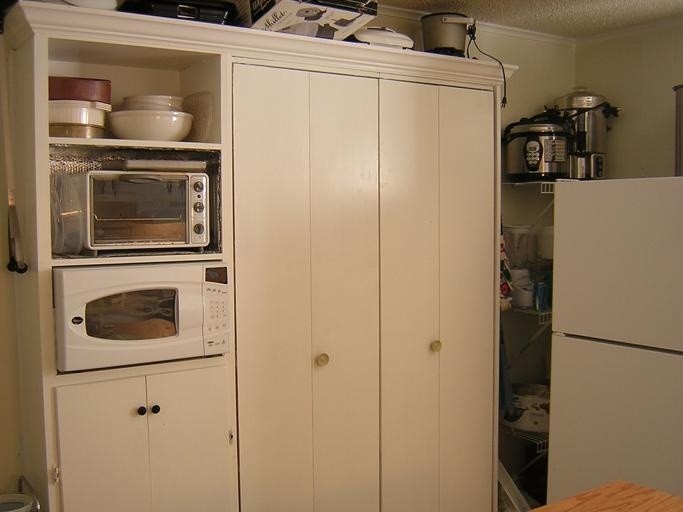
[502,115,569,180]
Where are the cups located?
[503,222,554,312]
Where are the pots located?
[420,11,472,58]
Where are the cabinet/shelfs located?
[377,76,495,512]
[227,60,379,512]
[17,365,235,511]
[8,29,227,264]
[500,176,577,456]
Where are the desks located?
[522,480,683,511]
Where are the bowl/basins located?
[501,381,549,433]
[48,74,193,141]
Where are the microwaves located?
[58,169,209,252]
[52,260,230,375]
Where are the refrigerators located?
[546,175,681,505]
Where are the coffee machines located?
[554,86,609,179]
